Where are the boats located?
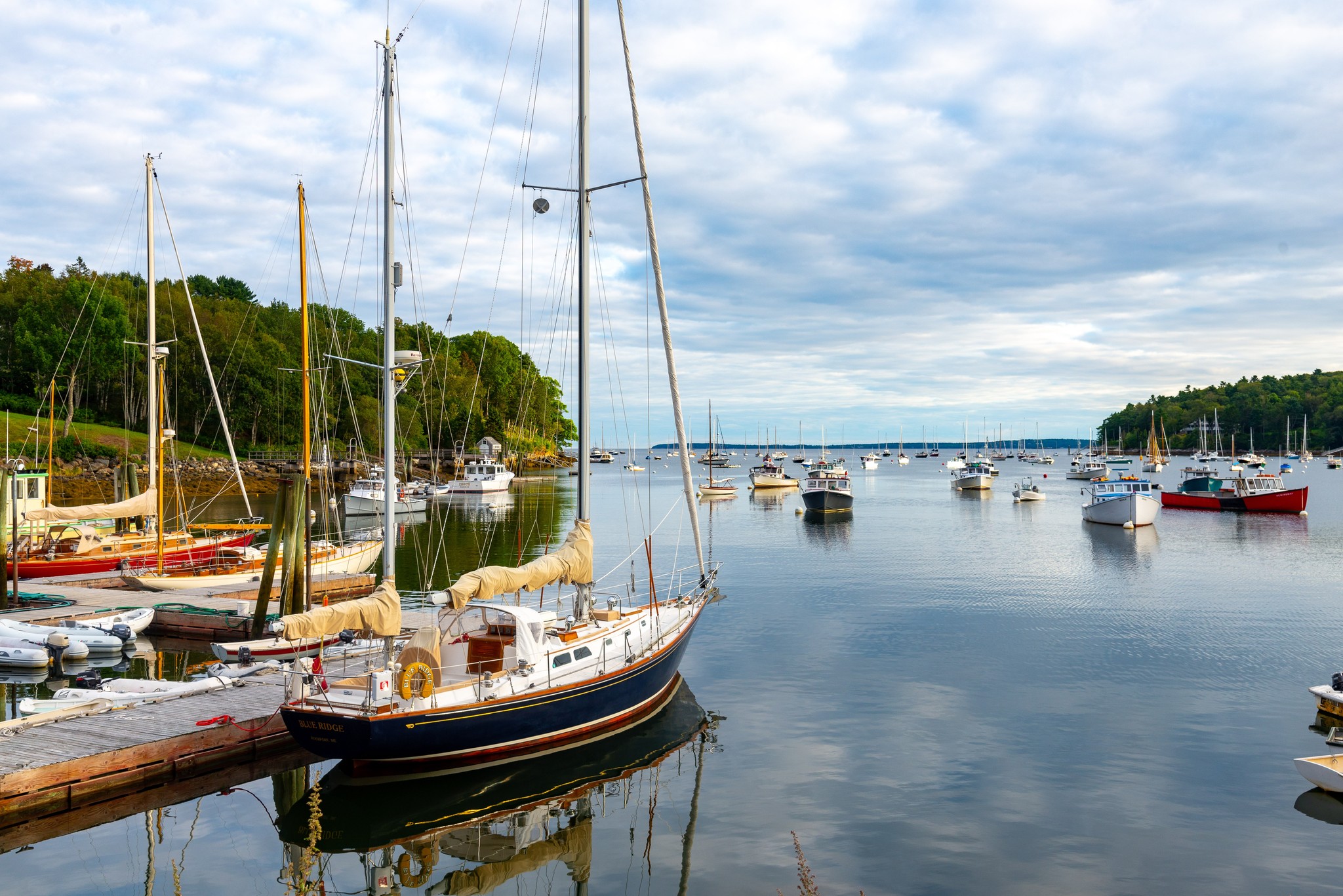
[0,608,344,714]
[749,423,799,488]
[1160,463,1309,514]
[1065,427,1110,479]
[1081,470,1161,528]
[117,177,383,595]
[277,0,722,784]
[697,399,739,495]
[1294,752,1343,795]
[343,455,514,517]
[1,154,265,593]
[799,424,854,514]
[948,414,994,490]
[1011,476,1045,502]
[588,413,1343,472]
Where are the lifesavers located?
[399,662,434,700]
[399,489,404,498]
